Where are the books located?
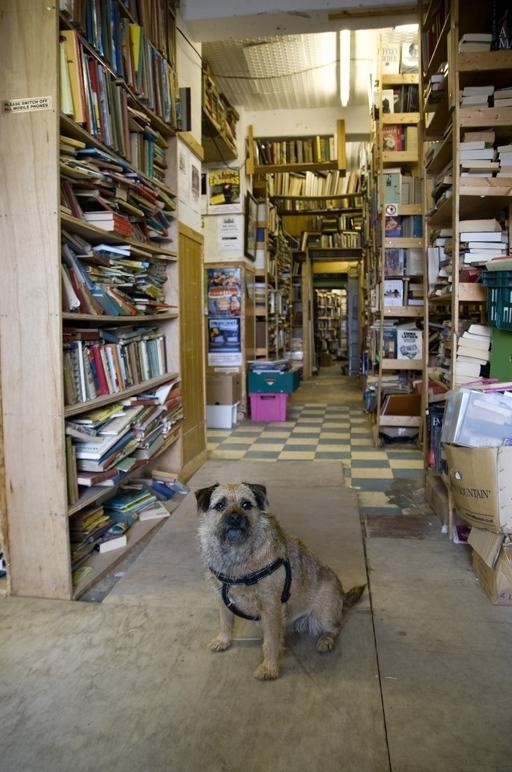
[361,30,511,473]
[58,2,192,571]
[203,72,372,374]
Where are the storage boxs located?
[206,372,242,405]
[248,363,303,393]
[467,526,512,606]
[249,394,292,422]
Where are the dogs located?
[194,481,369,681]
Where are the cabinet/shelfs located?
[368,0,510,538]
[0,0,180,603]
[247,135,369,382]
